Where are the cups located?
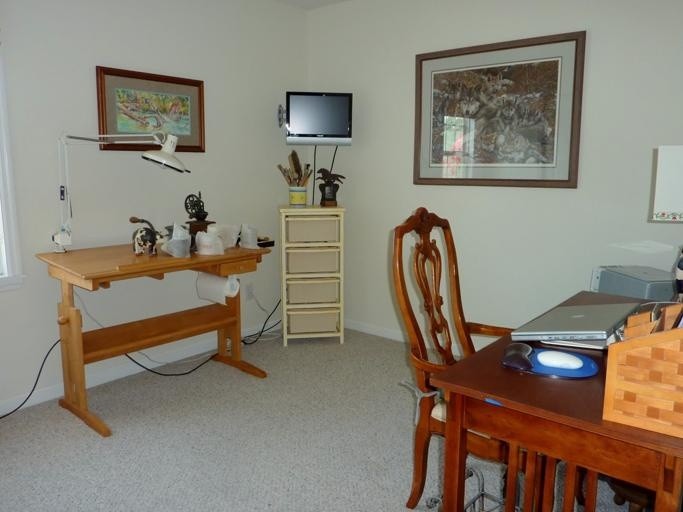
[287,187,307,207]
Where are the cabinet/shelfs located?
[277,206,345,350]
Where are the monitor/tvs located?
[286,91,353,145]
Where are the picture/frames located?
[95,65,206,153]
[412,29,586,191]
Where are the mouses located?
[537,351,583,369]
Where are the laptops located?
[510,303,640,342]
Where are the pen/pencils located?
[278,164,314,186]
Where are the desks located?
[33,235,272,432]
[430,286,683,512]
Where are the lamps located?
[650,146,683,303]
[50,131,191,253]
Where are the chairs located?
[391,206,580,511]
